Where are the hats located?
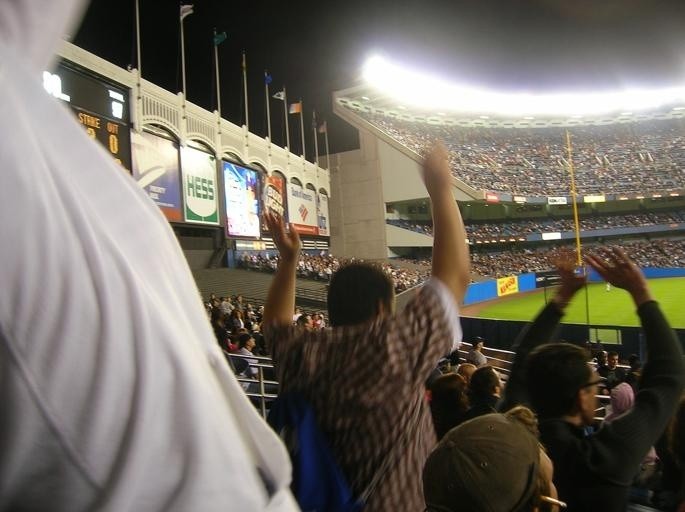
[421,409,538,512]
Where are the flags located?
[176,4,194,19]
[263,73,273,85]
[212,31,228,46]
[289,102,301,114]
[272,90,286,101]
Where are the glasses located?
[580,377,606,389]
[473,336,486,346]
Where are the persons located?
[203,114,684,512]
[0,1,311,512]
